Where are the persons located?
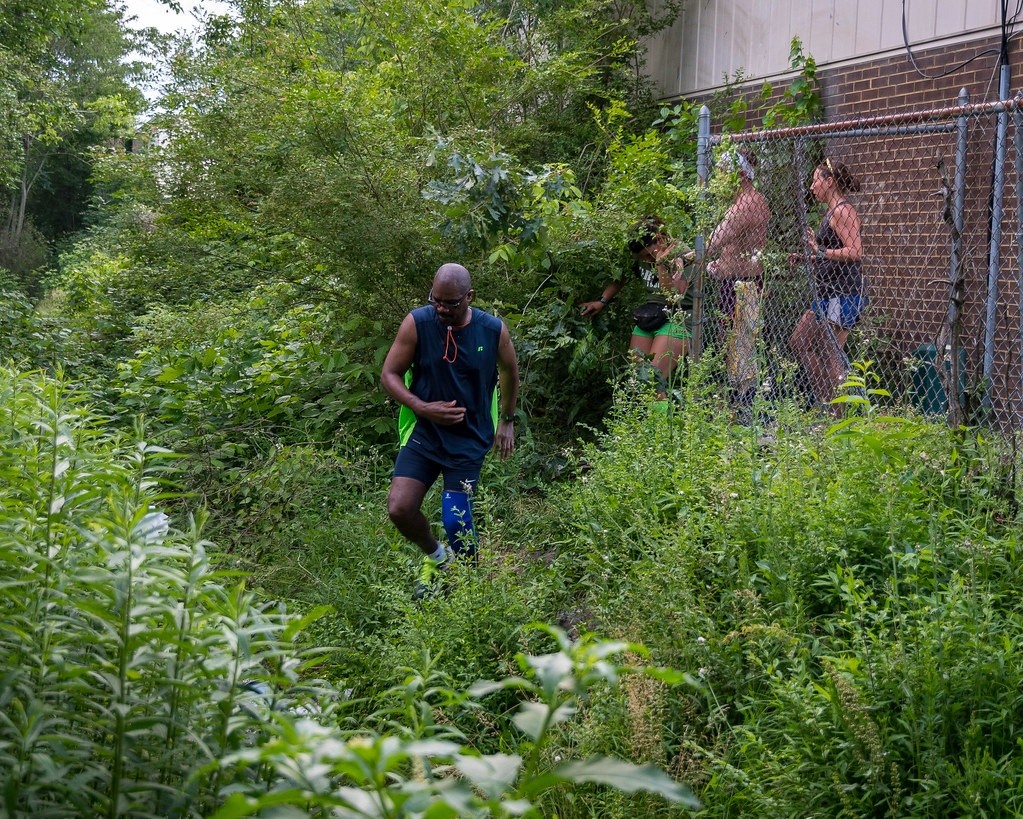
[582,222,693,380]
[380,263,519,598]
[665,144,772,427]
[790,159,866,414]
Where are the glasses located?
[822,157,835,179]
[428,286,471,310]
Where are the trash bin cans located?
[917,342,967,418]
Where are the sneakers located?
[412,550,450,599]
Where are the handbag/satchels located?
[632,303,672,332]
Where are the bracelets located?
[599,296,608,304]
